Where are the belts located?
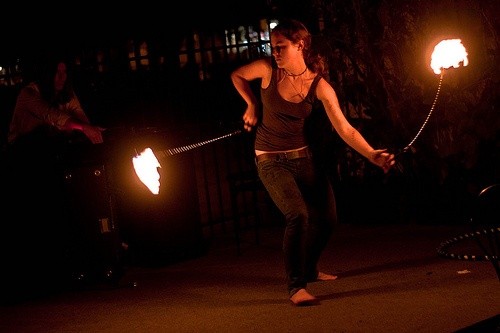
[257,147,310,160]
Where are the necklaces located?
[282,66,309,76]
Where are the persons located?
[6,60,107,144]
[231,20,396,306]
